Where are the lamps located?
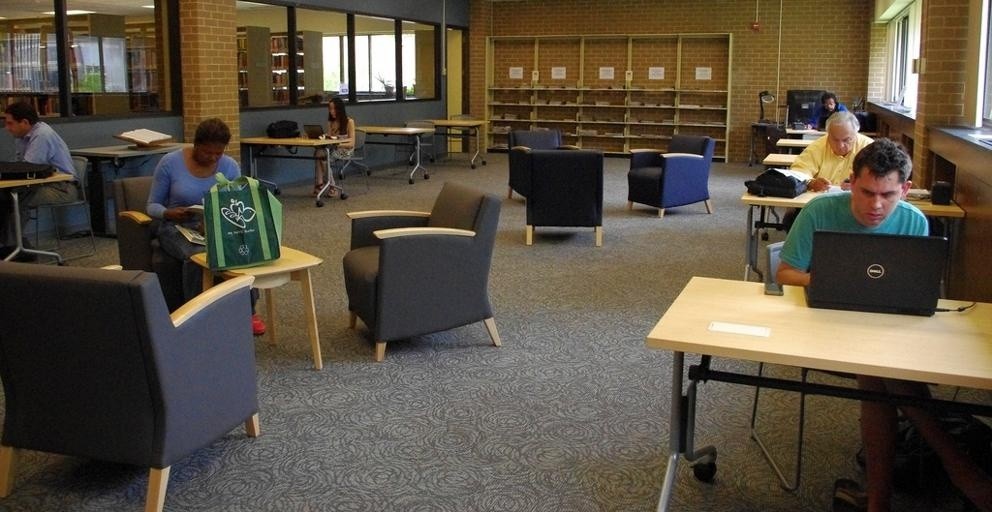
[759,90,775,120]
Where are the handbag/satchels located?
[203,172,282,271]
[745,170,808,198]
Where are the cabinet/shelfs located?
[236,25,271,108]
[1,16,159,122]
[482,32,733,163]
[271,30,324,102]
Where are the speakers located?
[932,180,952,205]
[854,110,877,131]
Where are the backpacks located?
[266,120,299,154]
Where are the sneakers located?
[251,313,266,335]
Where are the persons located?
[775,138,991,512]
[310,97,355,198]
[789,111,876,193]
[147,117,265,334]
[803,92,848,132]
[2,102,79,262]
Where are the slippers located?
[834,479,868,512]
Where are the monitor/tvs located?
[787,90,827,129]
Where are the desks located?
[70,143,186,170]
[242,119,491,206]
[648,275,987,509]
[743,127,964,298]
[189,247,328,368]
[0,170,73,265]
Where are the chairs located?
[750,240,913,492]
[627,134,708,212]
[27,155,98,255]
[0,256,264,511]
[507,127,560,197]
[113,174,158,274]
[343,183,501,363]
[524,147,604,249]
[856,112,878,132]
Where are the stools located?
[748,121,778,166]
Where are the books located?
[907,186,931,200]
[123,126,172,144]
[176,223,207,247]
[189,202,205,213]
[756,167,812,182]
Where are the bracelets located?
[807,178,816,190]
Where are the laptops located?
[304,125,324,139]
[805,230,949,317]
